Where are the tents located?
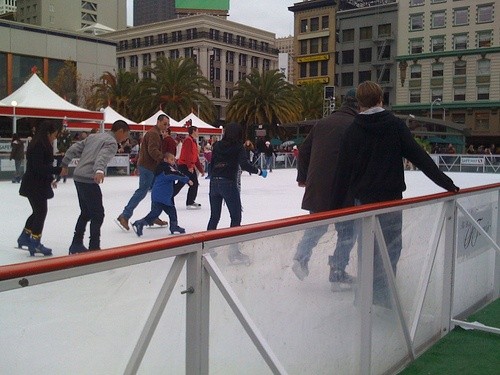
[0,73,223,154]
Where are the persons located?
[9,114,299,263]
[431,142,500,166]
[290,88,359,290]
[335,82,460,318]
